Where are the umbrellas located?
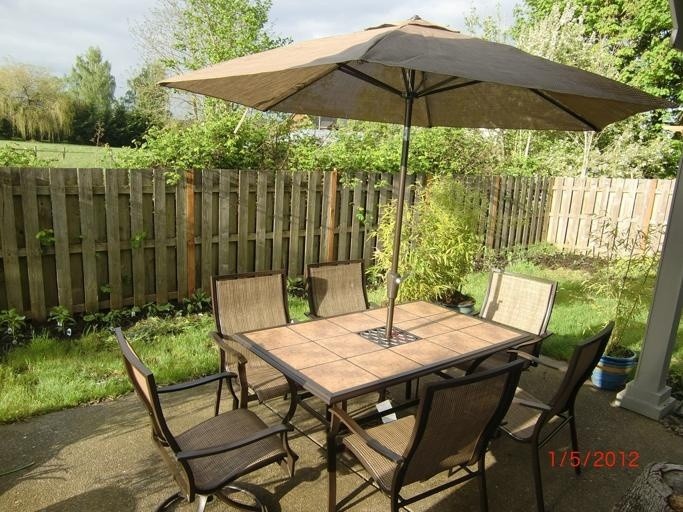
[155,14,679,339]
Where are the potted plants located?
[578,208,668,391]
[365,172,492,314]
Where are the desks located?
[232,300,543,511]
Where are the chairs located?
[496,322,618,511]
[336,359,524,511]
[210,270,302,417]
[116,326,299,512]
[454,268,559,375]
[302,260,374,320]
